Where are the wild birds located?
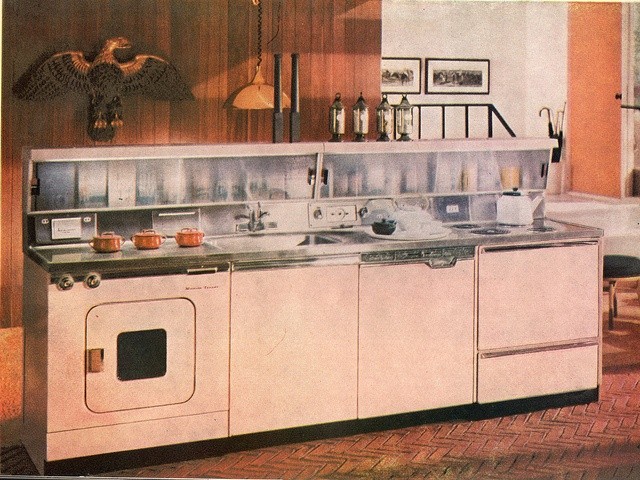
[16,37,194,141]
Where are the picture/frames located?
[380,57,422,96]
[425,57,491,95]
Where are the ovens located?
[47,261,229,463]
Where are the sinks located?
[203,231,345,254]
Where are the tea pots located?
[372,218,398,234]
[385,193,433,233]
[494,186,542,225]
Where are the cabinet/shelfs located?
[230,261,358,436]
[22,135,563,217]
[359,257,476,418]
[477,239,600,405]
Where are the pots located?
[130,229,167,249]
[174,228,205,247]
[88,231,125,253]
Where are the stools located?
[604,251,640,332]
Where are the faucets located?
[248,209,271,231]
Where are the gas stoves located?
[441,217,605,245]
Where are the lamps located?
[223,1,294,114]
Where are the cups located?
[429,220,442,234]
[500,168,520,189]
[459,168,476,193]
